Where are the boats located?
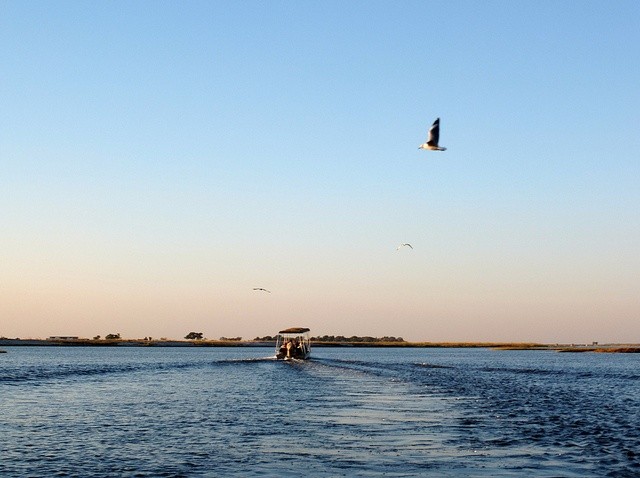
[276,327,312,361]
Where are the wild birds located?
[417,117,448,151]
[397,243,413,250]
[252,288,271,294]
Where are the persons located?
[278,336,308,358]
